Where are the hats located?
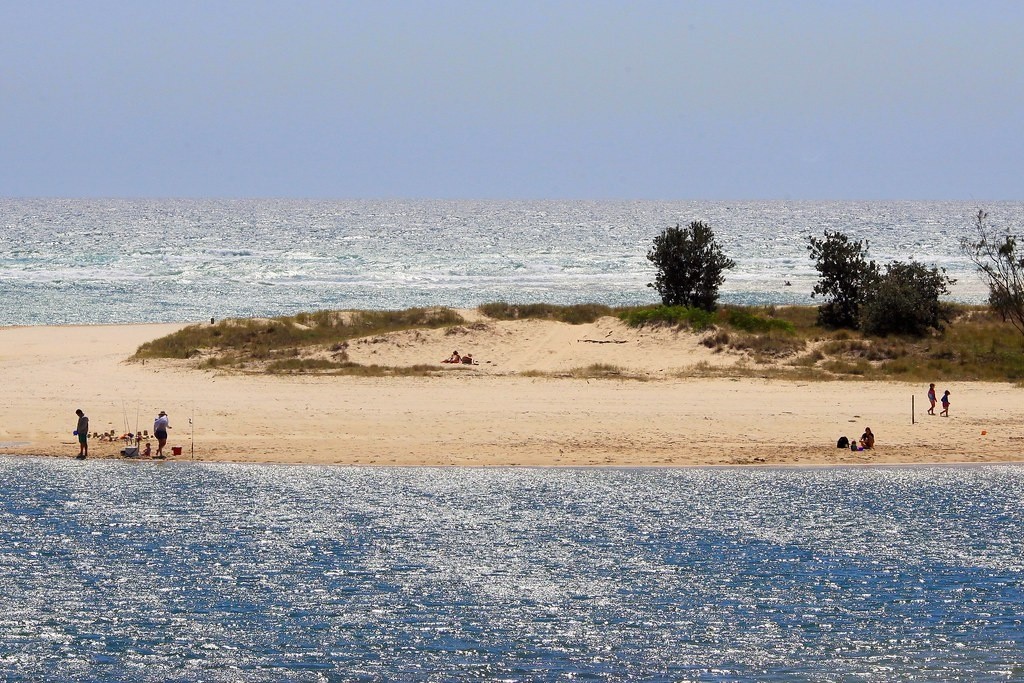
[453,351,458,354]
[158,411,168,416]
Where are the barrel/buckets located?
[172,446,182,455]
[858,446,863,450]
[982,430,986,435]
[125,447,138,458]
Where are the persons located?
[140,443,151,456]
[927,383,937,415]
[153,411,173,455]
[449,351,462,363]
[837,436,850,448]
[940,390,951,417]
[851,427,874,451]
[74,408,89,458]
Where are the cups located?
[73,431,77,435]
